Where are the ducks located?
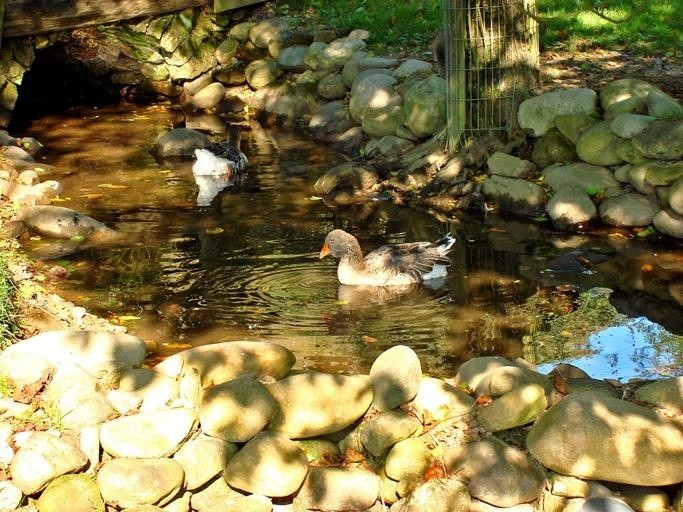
[192,143,247,175]
[319,229,456,286]
